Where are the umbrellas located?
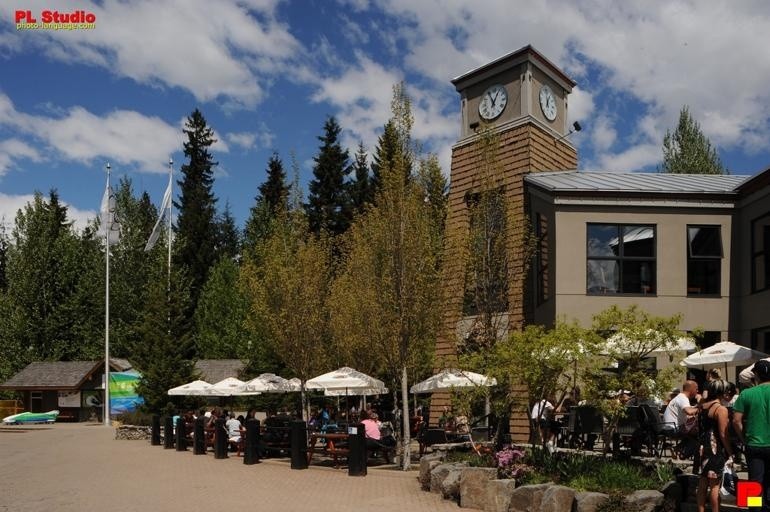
[532,327,770,399]
[168,366,389,422]
[410,367,498,393]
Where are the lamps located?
[552,121,582,143]
[468,122,483,135]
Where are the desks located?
[202,425,350,469]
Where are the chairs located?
[523,399,750,474]
[415,421,490,457]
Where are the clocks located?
[539,84,558,122]
[476,83,508,124]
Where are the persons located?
[412,406,469,441]
[532,360,770,512]
[173,407,382,464]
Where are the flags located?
[99,185,120,244]
[144,182,171,251]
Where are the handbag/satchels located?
[718,455,740,501]
